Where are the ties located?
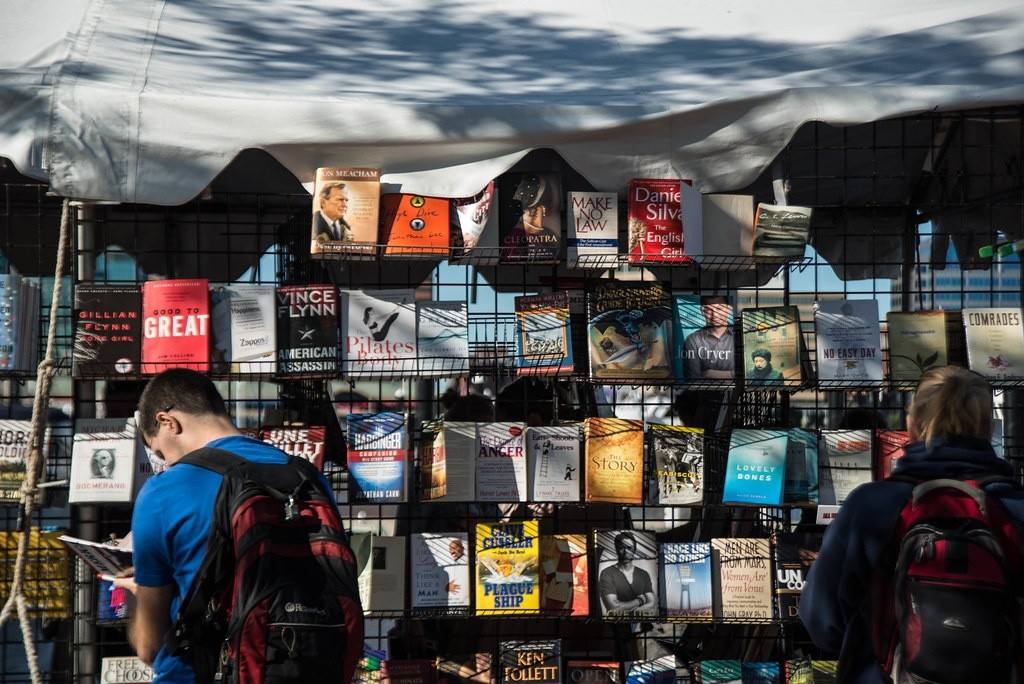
[331,222,339,241]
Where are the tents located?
[0,2,1024,276]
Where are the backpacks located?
[866,467,1024,683]
[164,448,366,684]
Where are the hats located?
[752,348,771,362]
[699,295,729,306]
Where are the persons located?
[797,367,1024,684]
[113,368,367,683]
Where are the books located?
[351,520,839,684]
[100,655,155,683]
[97,579,129,624]
[57,531,133,584]
[0,165,1013,508]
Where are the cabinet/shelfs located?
[0,167,1024,684]
